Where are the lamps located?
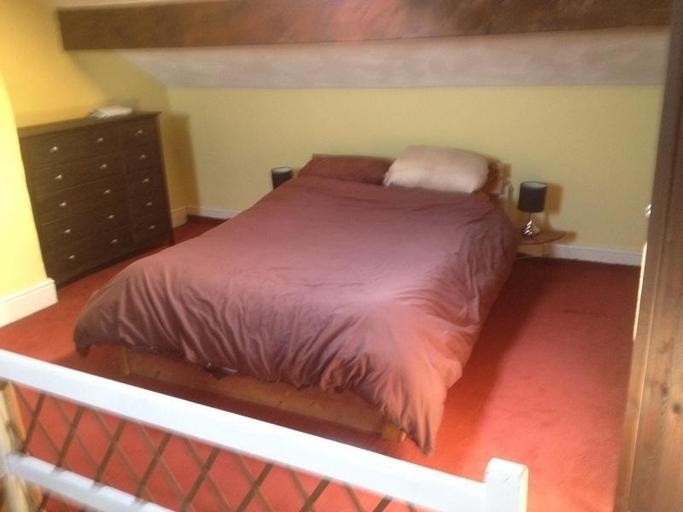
[271,166,294,191]
[518,183,548,236]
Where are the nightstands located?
[511,230,566,264]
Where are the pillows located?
[302,148,493,194]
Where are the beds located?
[78,163,519,445]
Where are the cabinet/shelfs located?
[17,109,176,287]
